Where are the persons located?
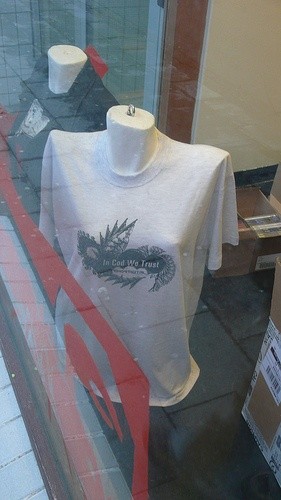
[43,105,239,408]
[6,45,116,224]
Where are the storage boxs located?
[205,187,281,279]
[241,257,281,488]
[268,164,281,213]
